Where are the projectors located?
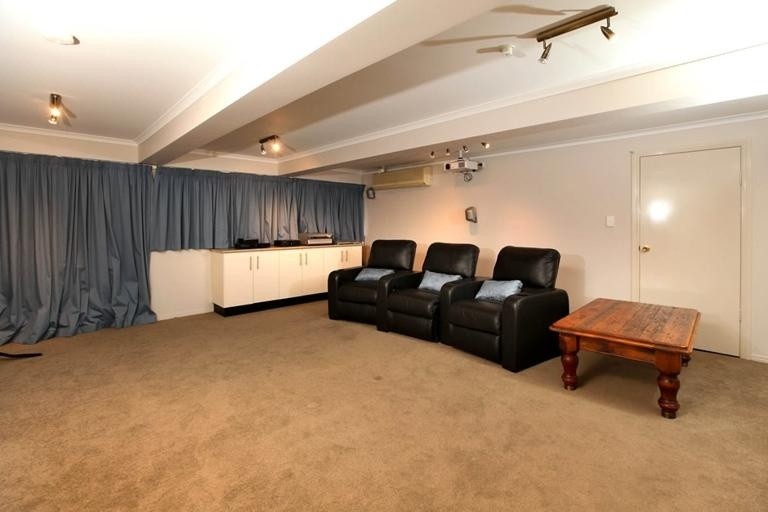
[443,161,478,173]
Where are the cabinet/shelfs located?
[325,246,362,293]
[279,249,325,302]
[224,251,280,309]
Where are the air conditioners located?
[370,167,432,190]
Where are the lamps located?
[535,7,619,64]
[46,93,62,125]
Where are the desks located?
[547,295,700,420]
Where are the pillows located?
[417,268,459,293]
[477,278,521,305]
[357,265,392,282]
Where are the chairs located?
[380,242,477,345]
[328,237,414,323]
[441,244,569,373]
